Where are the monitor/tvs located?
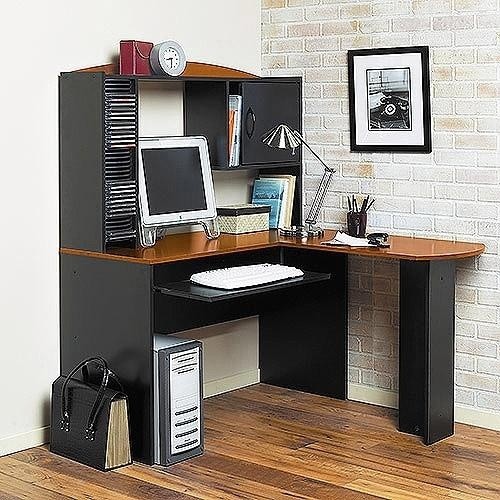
[137,135,220,248]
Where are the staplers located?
[367,233,390,248]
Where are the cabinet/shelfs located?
[178,72,306,174]
[56,69,144,255]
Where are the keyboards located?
[189,263,304,290]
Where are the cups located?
[346,212,368,237]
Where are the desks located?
[58,226,486,468]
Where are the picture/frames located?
[345,44,432,154]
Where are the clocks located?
[150,40,189,78]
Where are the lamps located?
[261,121,335,239]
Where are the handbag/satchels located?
[48,354,136,472]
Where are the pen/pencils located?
[348,195,375,213]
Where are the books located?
[252,174,297,229]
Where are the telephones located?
[378,96,409,122]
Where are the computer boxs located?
[153,333,205,466]
